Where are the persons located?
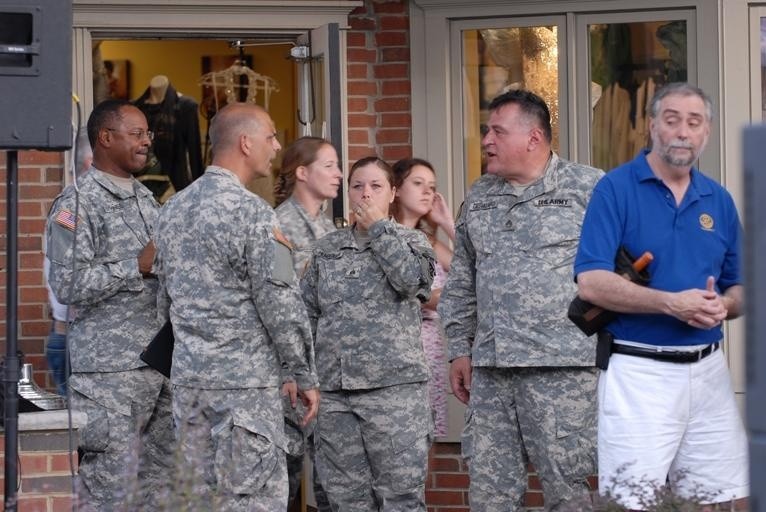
[46,97,177,512]
[569,79,759,512]
[437,88,610,512]
[151,101,323,512]
[300,156,440,512]
[130,72,205,197]
[270,134,338,512]
[42,258,71,400]
[383,158,462,439]
[102,61,123,101]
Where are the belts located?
[611,343,719,363]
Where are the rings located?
[357,209,361,214]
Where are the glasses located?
[108,128,155,141]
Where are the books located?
[138,319,174,380]
